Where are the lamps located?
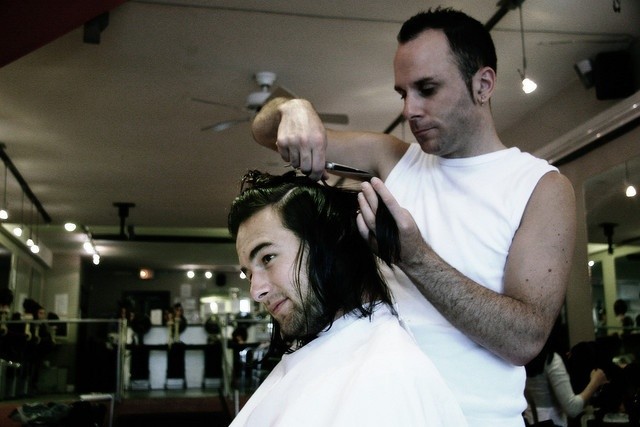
[518,4,538,96]
[26,203,34,246]
[13,190,25,236]
[623,161,637,200]
[0,165,9,219]
[30,210,40,254]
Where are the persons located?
[228,169,467,427]
[0,288,54,397]
[525,313,606,427]
[253,6,577,427]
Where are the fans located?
[190,71,350,134]
